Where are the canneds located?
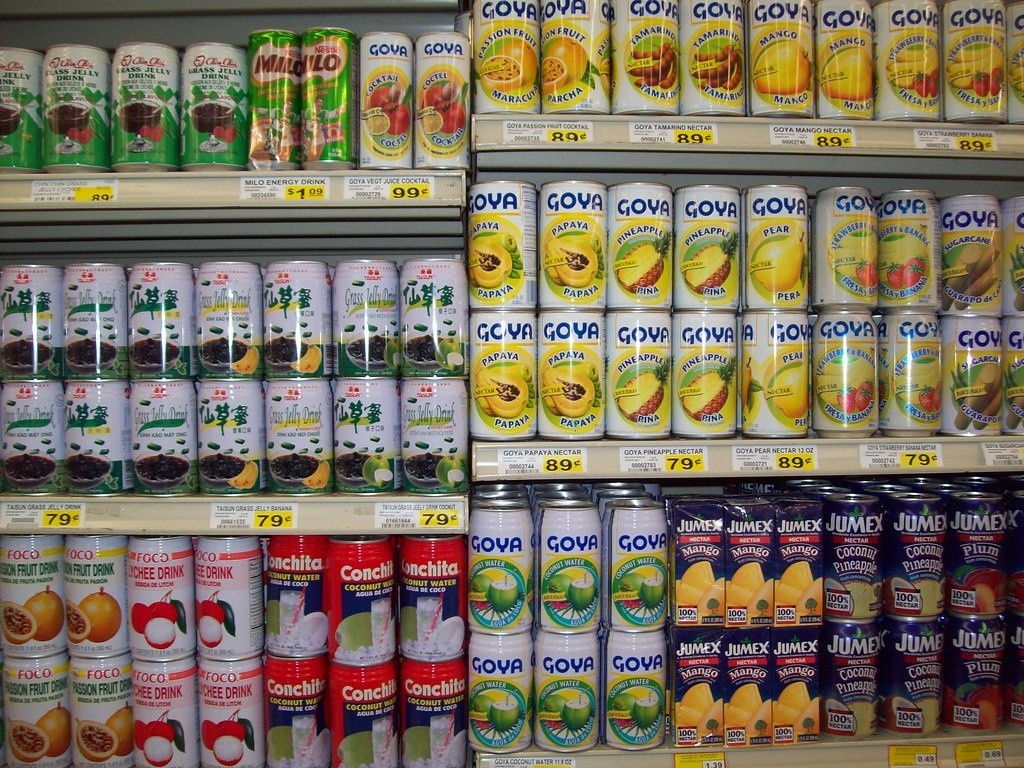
[0,1,1024,768]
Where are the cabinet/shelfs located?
[0,0,1022,766]
